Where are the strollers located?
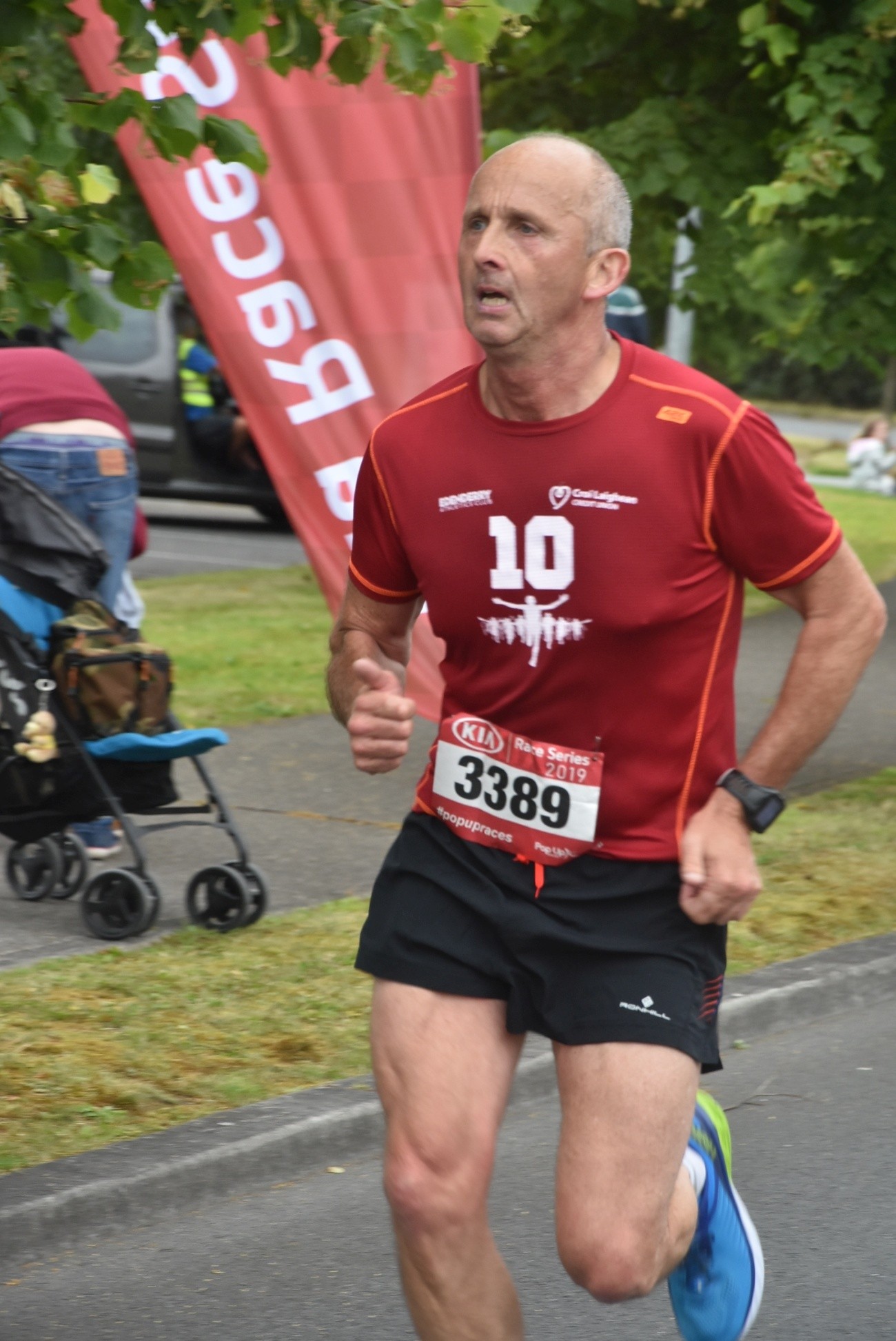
[1,462,274,940]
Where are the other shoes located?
[75,823,124,858]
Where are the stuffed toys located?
[14,712,58,763]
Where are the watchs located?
[715,768,786,834]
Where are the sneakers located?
[666,1089,765,1339]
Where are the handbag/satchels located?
[47,598,173,737]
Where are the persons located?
[844,413,896,495]
[176,306,265,474]
[325,127,885,1341]
[0,347,149,856]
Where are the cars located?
[46,266,293,531]
